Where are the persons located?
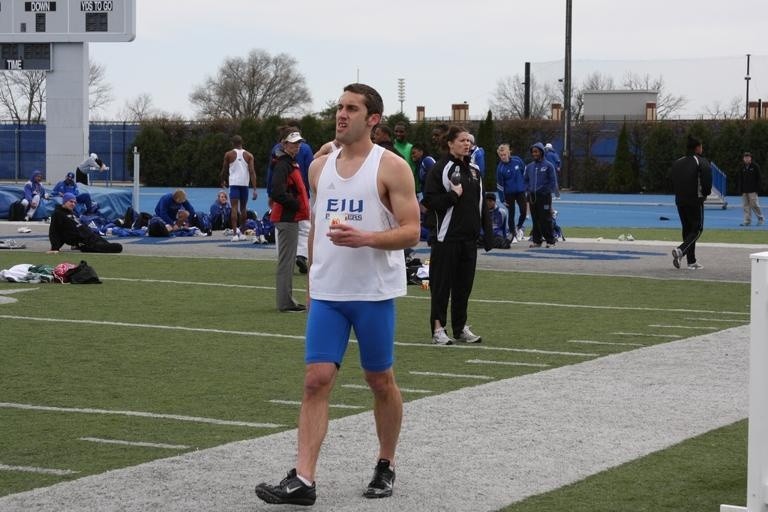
[22,122,565,252]
[739,151,765,226]
[268,126,310,313]
[672,136,712,270]
[421,125,493,345]
[255,84,422,506]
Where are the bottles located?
[451,166,463,186]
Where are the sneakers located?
[285,305,306,312]
[454,325,482,343]
[254,468,316,506]
[511,236,518,244]
[686,262,703,269]
[672,248,683,268]
[516,225,524,241]
[365,458,396,499]
[231,234,267,244]
[431,327,453,345]
[295,257,308,273]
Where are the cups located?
[421,279,430,290]
[327,212,347,233]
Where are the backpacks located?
[8,201,25,221]
[122,207,168,236]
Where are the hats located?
[281,131,304,143]
[544,142,552,149]
[485,193,496,202]
[62,192,76,203]
[67,173,73,178]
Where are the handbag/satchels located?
[0,260,103,286]
